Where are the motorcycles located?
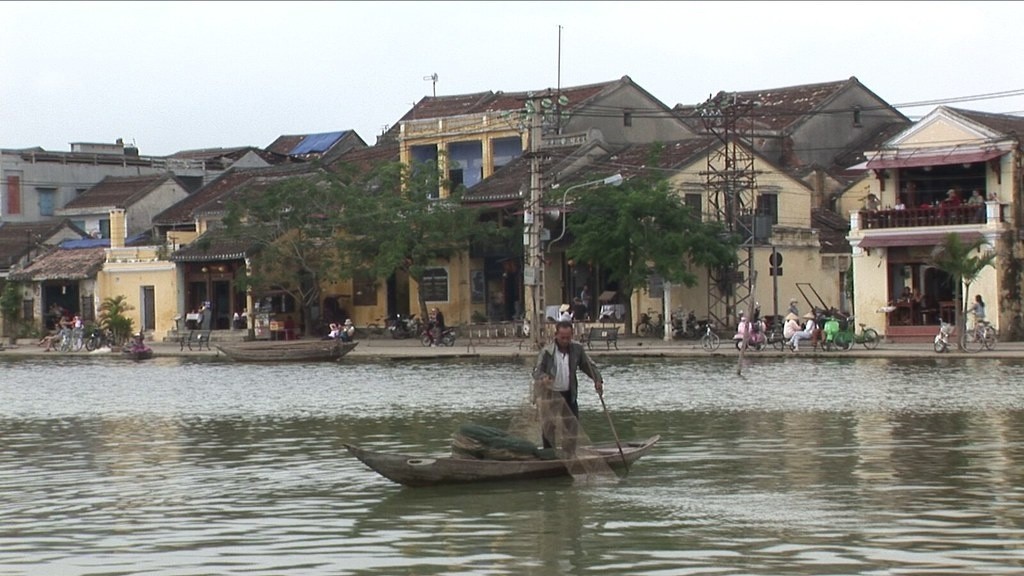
[420,318,456,347]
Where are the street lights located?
[524,174,623,338]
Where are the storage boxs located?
[270,321,285,331]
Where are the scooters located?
[366,312,437,340]
[933,318,952,353]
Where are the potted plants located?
[97,295,133,353]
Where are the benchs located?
[579,327,621,351]
[180,329,213,351]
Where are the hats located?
[789,298,798,305]
[344,319,352,325]
[784,312,799,320]
[803,312,815,319]
[559,303,570,312]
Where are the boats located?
[343,432,663,484]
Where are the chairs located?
[195,314,205,330]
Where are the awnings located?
[846,150,1011,170]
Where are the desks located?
[276,328,301,340]
[186,313,202,330]
[599,304,626,322]
[545,305,571,323]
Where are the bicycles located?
[960,321,998,354]
[634,304,881,352]
[57,325,115,352]
[966,311,996,344]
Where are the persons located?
[430,307,444,346]
[37,302,85,351]
[677,304,688,333]
[283,316,300,340]
[783,298,820,352]
[967,294,986,342]
[901,286,923,324]
[322,319,355,342]
[867,189,983,227]
[532,321,604,448]
[558,285,592,335]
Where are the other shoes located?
[785,342,793,346]
[792,348,799,352]
